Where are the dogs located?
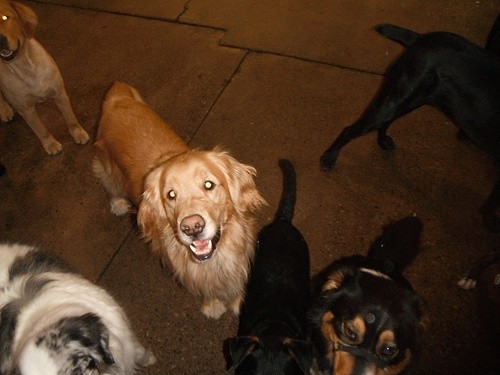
[318,23,500,290]
[221,159,314,375]
[0,241,157,374]
[310,216,424,375]
[90,82,271,319]
[0,0,90,156]
